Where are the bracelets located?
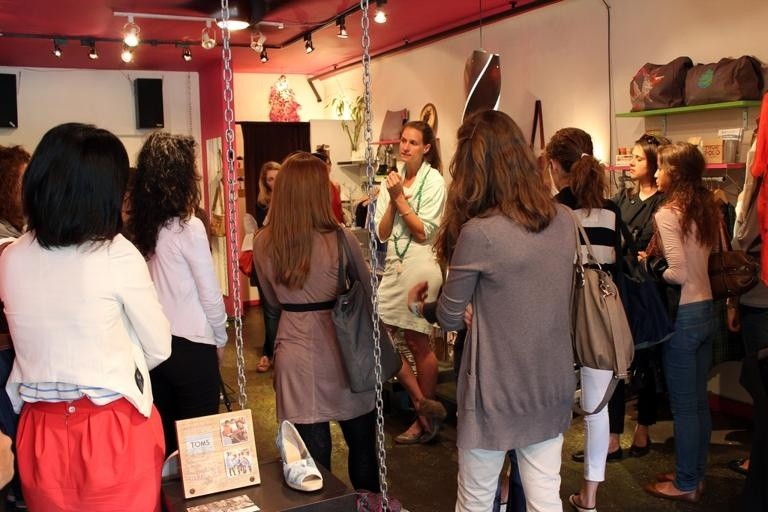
[399,208,414,218]
[411,301,423,320]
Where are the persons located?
[637,141,731,504]
[432,108,582,512]
[130,132,229,424]
[0,144,34,238]
[374,121,451,445]
[253,153,380,495]
[574,133,673,463]
[545,124,634,512]
[727,182,768,475]
[251,161,281,374]
[312,153,352,227]
[4,118,176,512]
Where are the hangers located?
[617,176,726,198]
[725,169,742,196]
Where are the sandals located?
[256,358,270,372]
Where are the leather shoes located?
[656,471,706,492]
[573,447,624,461]
[729,456,748,473]
[644,481,699,502]
[628,435,651,458]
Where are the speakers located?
[0,73,19,130]
[134,77,167,131]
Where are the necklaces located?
[393,163,432,274]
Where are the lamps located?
[121,22,141,48]
[201,27,216,50]
[121,47,134,65]
[214,2,250,32]
[336,17,349,39]
[304,33,315,54]
[260,52,270,64]
[51,44,63,58]
[182,47,192,62]
[373,1,386,24]
[89,45,99,60]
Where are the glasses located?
[641,134,659,146]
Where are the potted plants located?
[325,94,366,161]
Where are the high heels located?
[394,421,422,443]
[416,398,448,442]
[276,420,323,492]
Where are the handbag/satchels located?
[528,99,551,190]
[683,54,764,106]
[331,225,404,394]
[612,208,667,343]
[708,218,759,298]
[630,56,692,112]
[558,202,635,416]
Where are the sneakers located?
[568,493,596,512]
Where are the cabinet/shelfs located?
[610,100,761,174]
[337,161,371,211]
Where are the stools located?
[161,455,362,512]
